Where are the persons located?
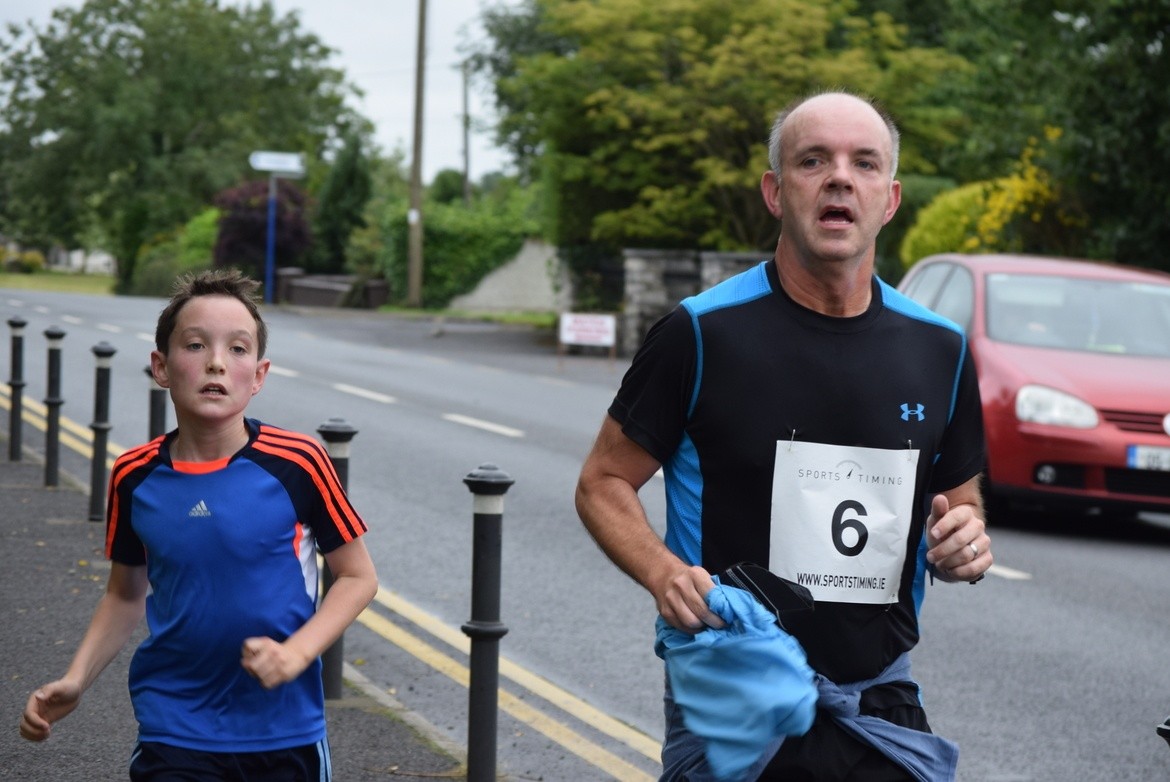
[18,268,378,782]
[574,88,994,782]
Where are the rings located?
[967,543,981,561]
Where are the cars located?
[889,250,1169,529]
